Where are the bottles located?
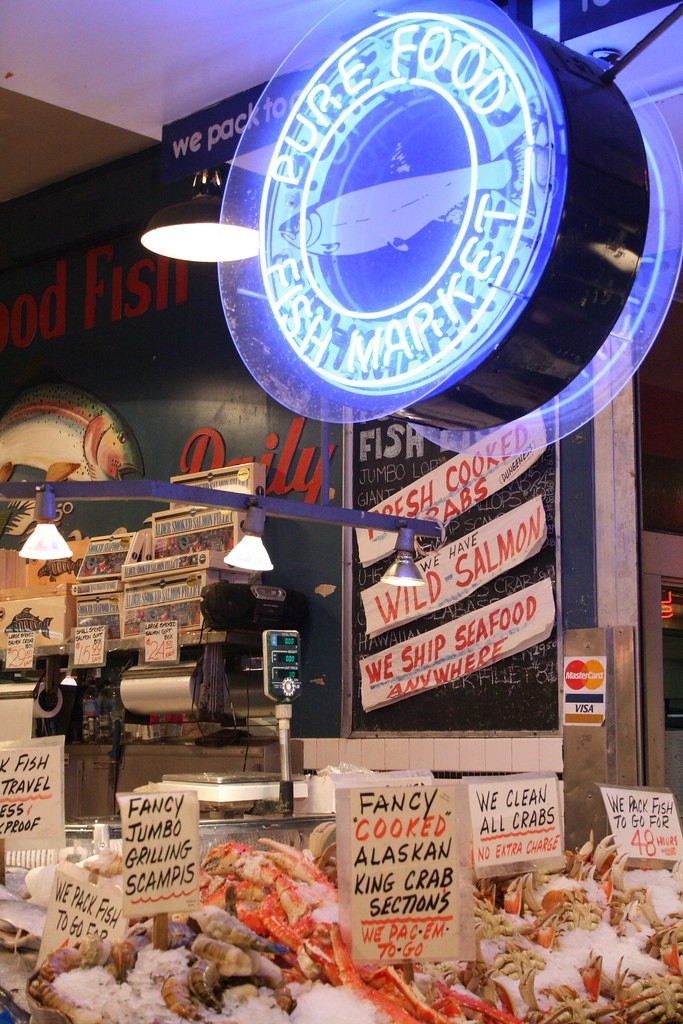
[82,679,97,742]
[97,689,113,741]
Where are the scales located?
[133,629,309,818]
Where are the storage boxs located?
[0,462,266,648]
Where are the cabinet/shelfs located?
[64,738,303,820]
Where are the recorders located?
[201,581,310,633]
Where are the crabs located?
[29,821,683,1024]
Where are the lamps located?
[139,168,258,262]
[224,498,273,572]
[379,518,425,586]
[18,485,73,561]
